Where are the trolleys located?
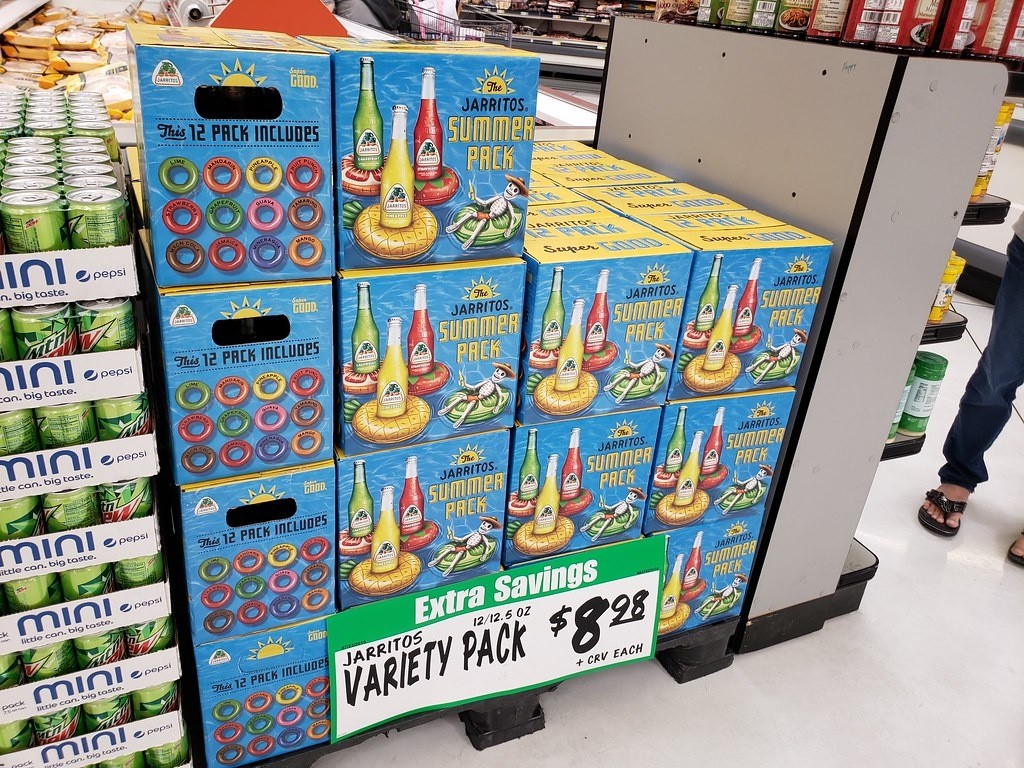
[392,0,515,50]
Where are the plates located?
[911,21,976,46]
[779,9,810,31]
[677,1,699,15]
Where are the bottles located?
[926,251,966,326]
[885,351,948,444]
[969,100,1017,203]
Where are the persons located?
[919,212,1024,568]
[458,0,483,7]
[325,0,397,34]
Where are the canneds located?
[883,103,1014,443]
[1,86,191,768]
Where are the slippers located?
[917,481,972,535]
[1007,530,1024,565]
